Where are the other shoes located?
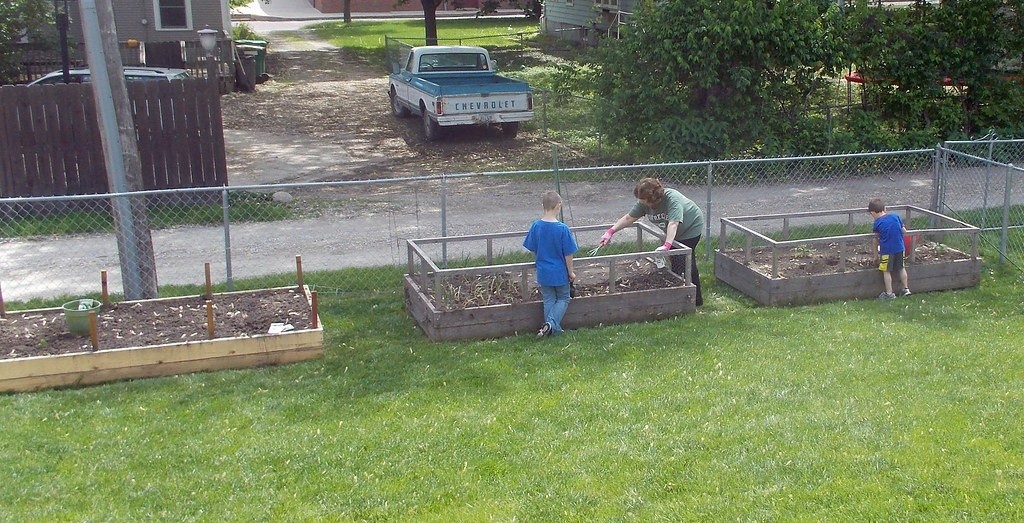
[899,288,912,297]
[879,291,896,298]
[538,322,551,338]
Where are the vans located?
[16,66,194,153]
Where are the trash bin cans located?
[235,44,266,84]
[234,57,256,93]
[234,39,270,84]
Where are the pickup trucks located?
[389,45,533,140]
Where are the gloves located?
[655,242,671,252]
[599,229,616,244]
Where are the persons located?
[868,198,911,300]
[598,178,705,306]
[523,190,579,339]
